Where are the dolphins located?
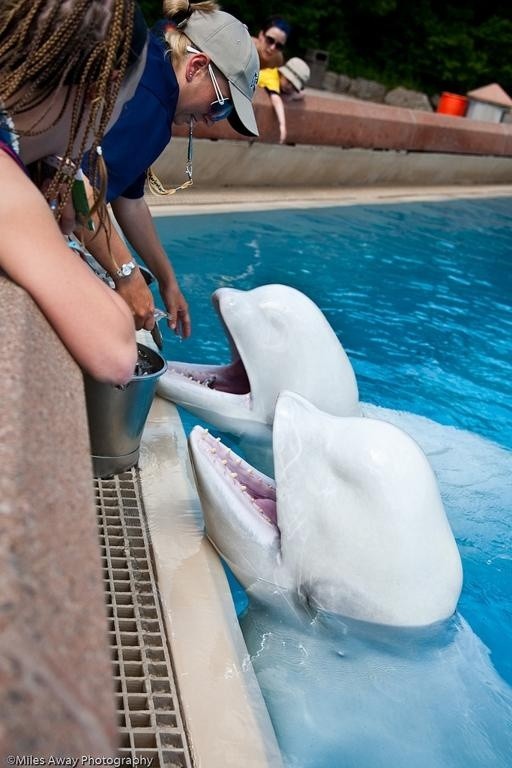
[186,387,512,768]
[132,282,512,565]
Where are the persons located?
[257,56,312,145]
[0,0,151,387]
[251,18,290,69]
[56,0,261,340]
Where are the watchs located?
[111,258,136,280]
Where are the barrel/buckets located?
[79,249,156,295]
[81,342,170,477]
[436,92,470,117]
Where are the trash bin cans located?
[306,49,330,89]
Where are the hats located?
[278,57,311,94]
[177,9,259,140]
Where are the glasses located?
[264,33,285,53]
[186,45,232,122]
